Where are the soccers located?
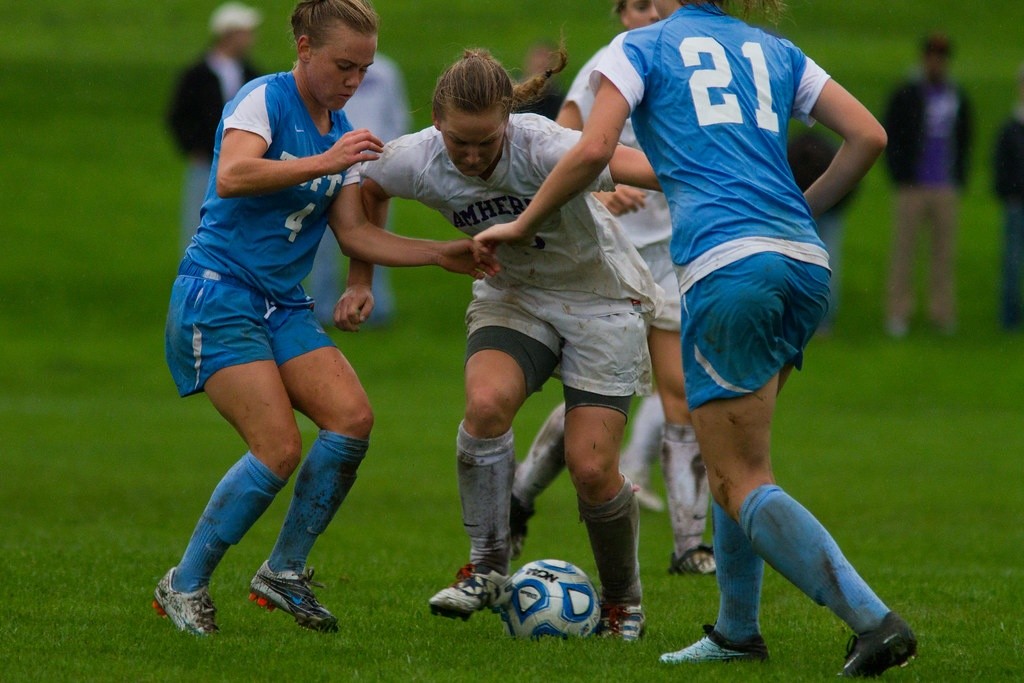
[504,559,602,639]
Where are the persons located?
[152,0,502,638]
[509,0,918,678]
[165,0,414,327]
[881,33,1024,338]
[333,42,665,643]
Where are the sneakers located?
[428,563,513,621]
[659,624,767,664]
[842,612,919,678]
[671,544,715,575]
[596,595,644,642]
[153,568,219,635]
[247,559,338,633]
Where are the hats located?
[211,1,261,39]
